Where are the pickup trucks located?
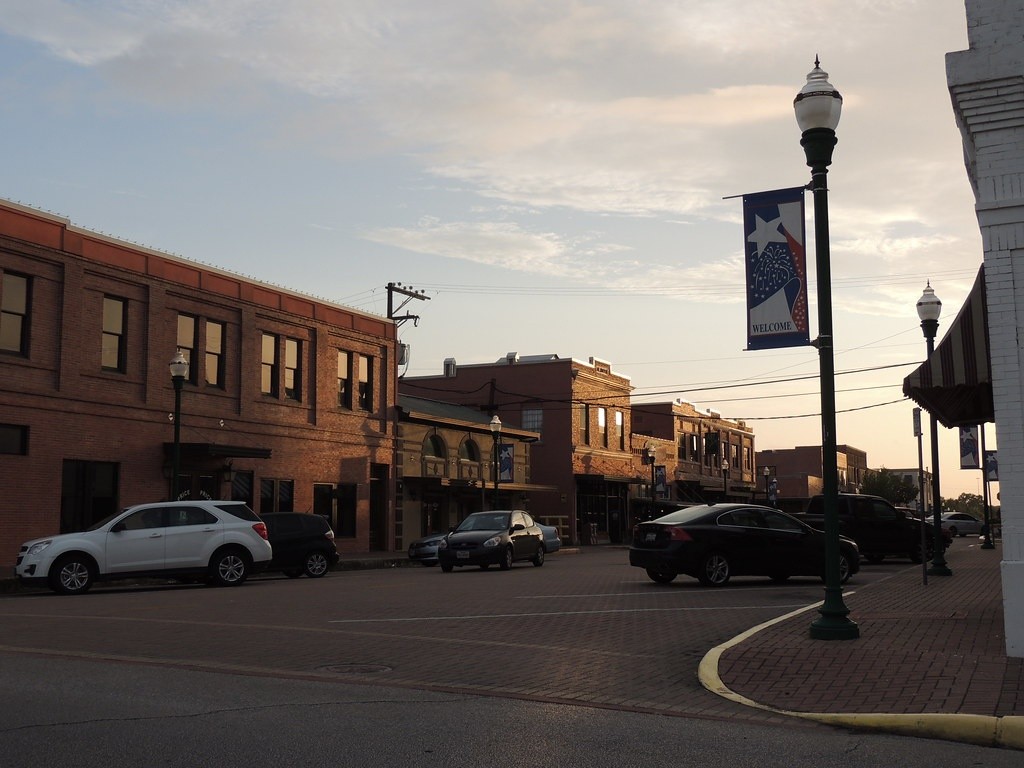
[780,492,954,564]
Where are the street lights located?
[790,52,865,639]
[647,441,659,519]
[165,342,191,502]
[762,465,770,506]
[912,278,954,578]
[721,459,729,503]
[489,414,504,510]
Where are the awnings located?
[903,263,995,429]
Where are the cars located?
[259,512,341,578]
[407,519,561,567]
[437,509,545,574]
[895,506,936,527]
[14,501,274,593]
[777,499,811,515]
[628,501,861,589]
[923,511,984,536]
